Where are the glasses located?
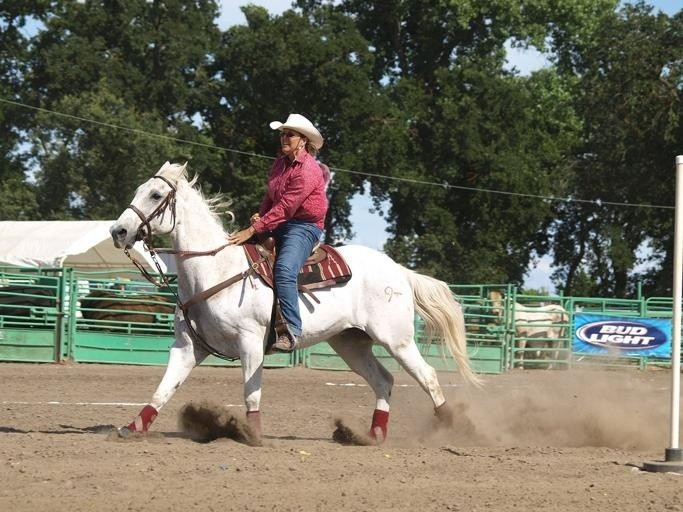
[279,130,299,140]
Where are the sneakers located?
[272,334,303,351]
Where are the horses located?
[484,286,574,369]
[106,157,490,447]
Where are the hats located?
[270,114,324,149]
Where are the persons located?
[226,114,329,352]
